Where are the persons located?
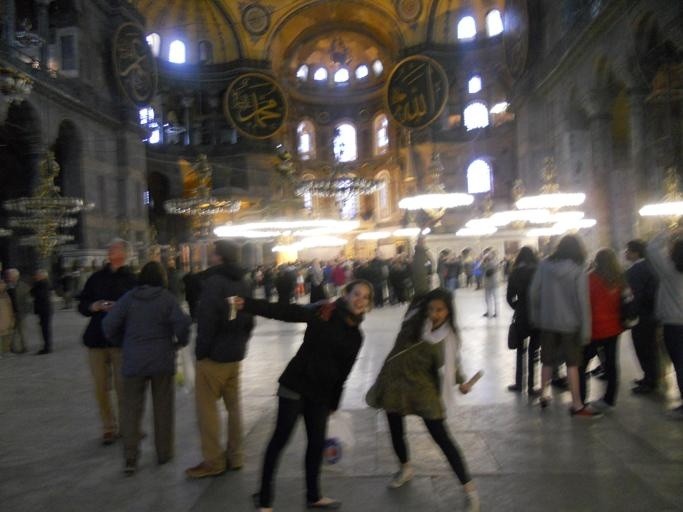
[528,234,605,420]
[186,240,255,478]
[29,269,53,354]
[480,245,500,319]
[101,261,191,474]
[645,214,683,412]
[252,248,481,306]
[506,246,540,395]
[76,239,147,443]
[6,269,30,354]
[234,279,374,512]
[623,239,658,396]
[365,230,479,512]
[578,248,622,408]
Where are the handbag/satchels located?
[319,411,343,466]
[171,348,191,389]
[615,276,641,331]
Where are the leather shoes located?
[251,493,270,511]
[308,498,343,511]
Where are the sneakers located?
[571,405,604,420]
[123,457,138,473]
[465,491,481,511]
[540,395,553,412]
[386,469,412,490]
[102,432,119,442]
[187,461,224,478]
